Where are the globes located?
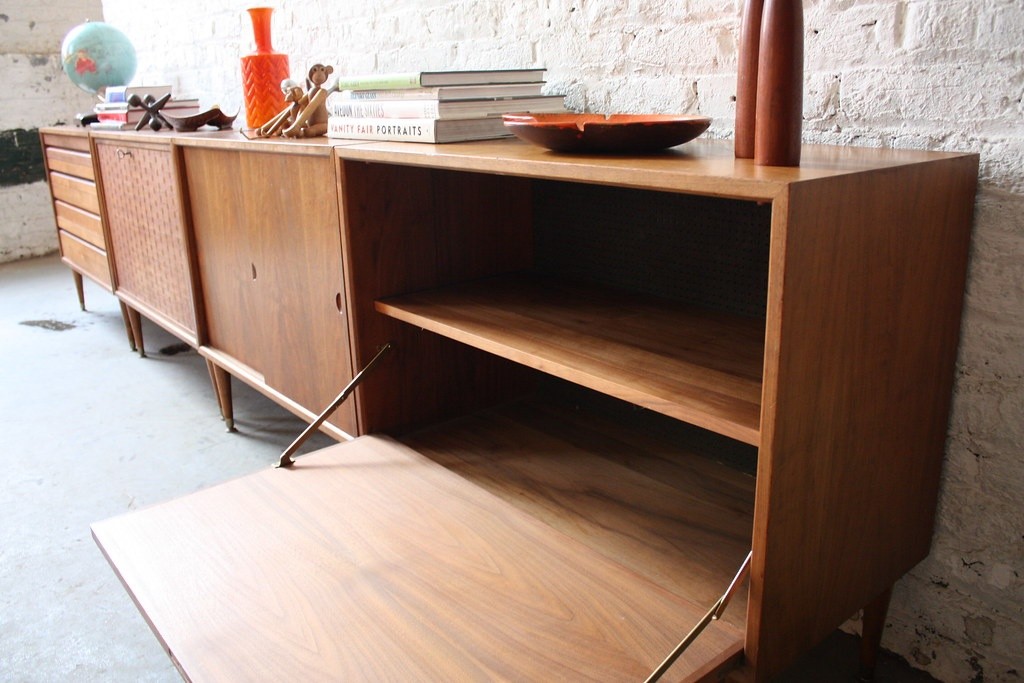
[59,19,137,127]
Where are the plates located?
[159,107,241,132]
[500,112,714,155]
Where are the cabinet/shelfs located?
[37,123,180,351]
[88,137,981,683]
[170,130,360,442]
[86,130,263,421]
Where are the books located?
[326,67,568,144]
[89,84,202,131]
